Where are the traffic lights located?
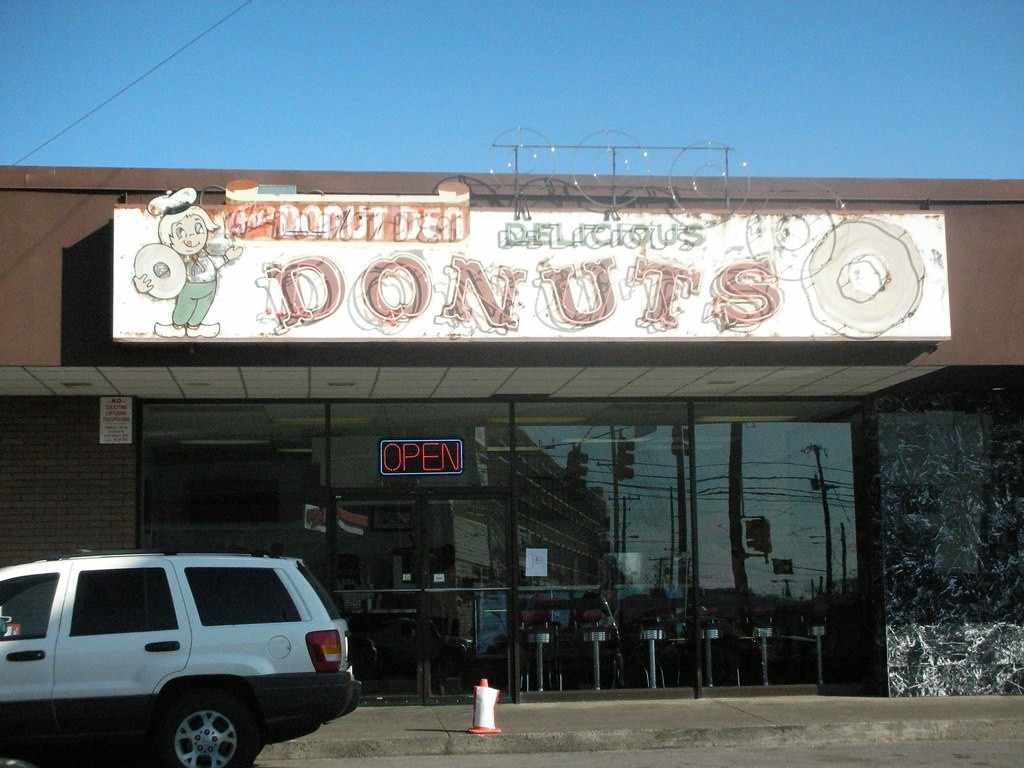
[564,442,589,497]
[615,436,635,481]
[671,426,693,457]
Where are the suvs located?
[0,548,363,768]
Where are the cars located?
[344,585,763,691]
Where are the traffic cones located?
[467,678,502,734]
